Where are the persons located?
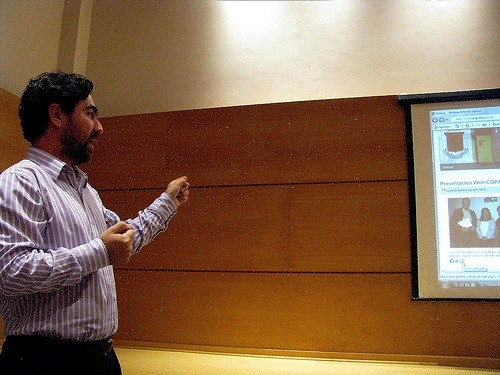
[474,206,495,242]
[494,206,500,244]
[448,197,476,246]
[0,66,195,372]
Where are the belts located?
[4,335,113,356]
